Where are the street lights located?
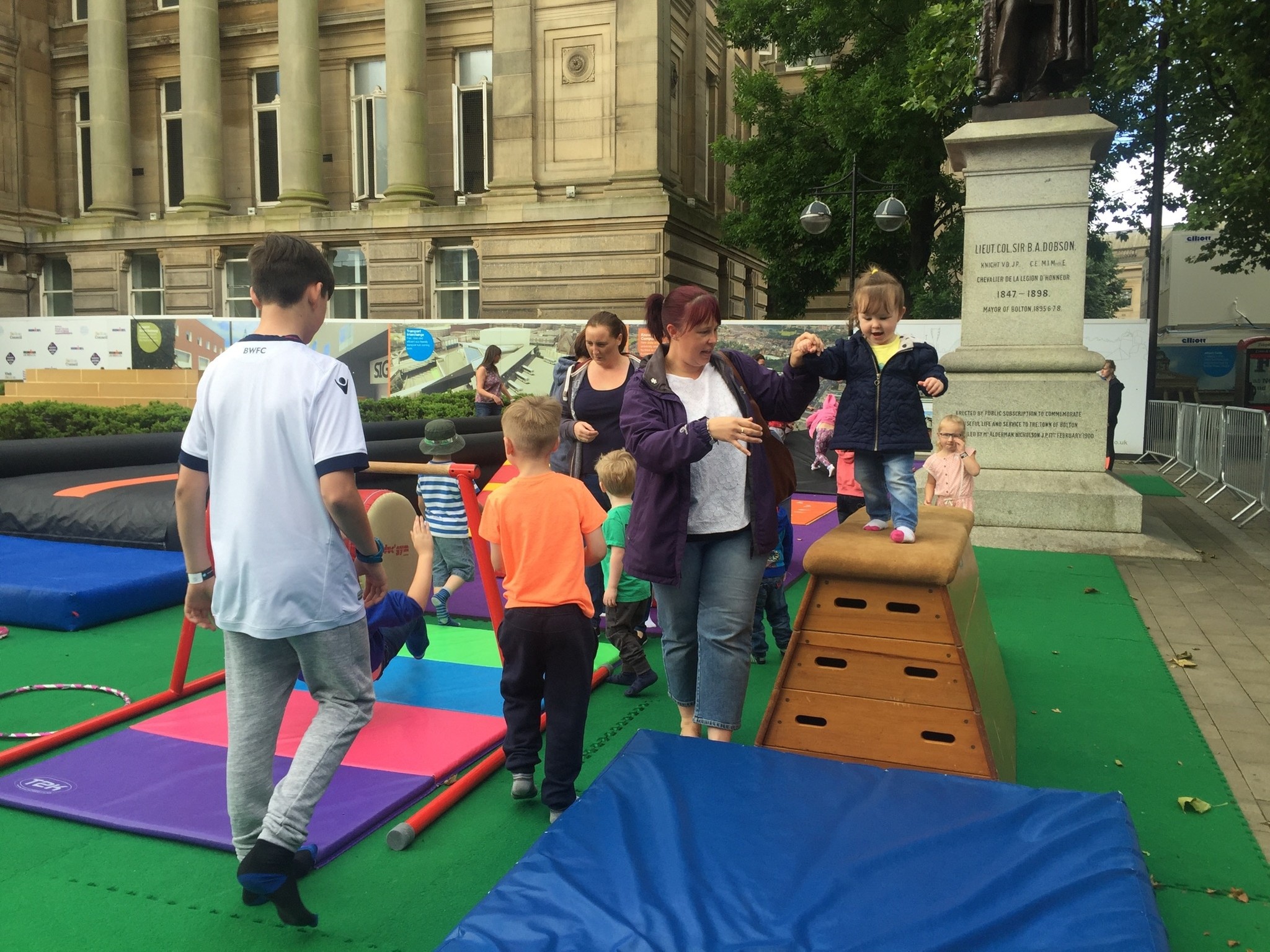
[800,153,909,337]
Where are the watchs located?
[355,537,384,564]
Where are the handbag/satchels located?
[764,435,796,505]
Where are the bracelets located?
[960,451,968,458]
[706,419,713,440]
[186,565,214,585]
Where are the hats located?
[418,419,465,455]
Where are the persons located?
[549,283,866,744]
[924,415,980,512]
[798,263,949,544]
[1101,360,1126,472]
[417,421,483,626]
[477,393,609,824]
[175,233,389,925]
[362,514,435,682]
[475,345,515,418]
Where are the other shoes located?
[636,630,648,645]
[595,627,600,638]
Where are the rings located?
[741,427,743,434]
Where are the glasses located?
[940,433,962,438]
